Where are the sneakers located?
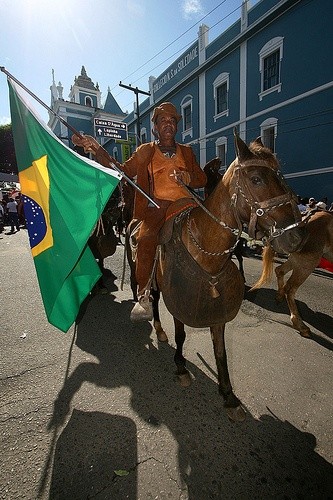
[130,301,154,322]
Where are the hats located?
[315,201,326,209]
[151,102,182,122]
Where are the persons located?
[0,191,20,232]
[72,101,207,323]
[297,194,327,218]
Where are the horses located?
[125,128,309,423]
[248,209,333,338]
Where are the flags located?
[7,77,122,335]
